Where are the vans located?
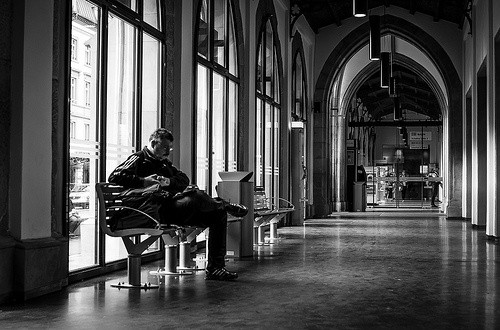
[69,184,89,209]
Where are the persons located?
[107,129,247,281]
[399,170,409,202]
[383,169,395,199]
[431,168,440,207]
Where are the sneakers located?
[205,267,238,280]
[227,203,248,217]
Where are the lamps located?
[380,35,390,89]
[393,100,409,147]
[389,66,396,97]
[353,0,367,17]
[368,0,381,61]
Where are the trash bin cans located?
[216,171,257,259]
[354,181,367,212]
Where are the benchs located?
[95,182,210,290]
[253,195,296,247]
